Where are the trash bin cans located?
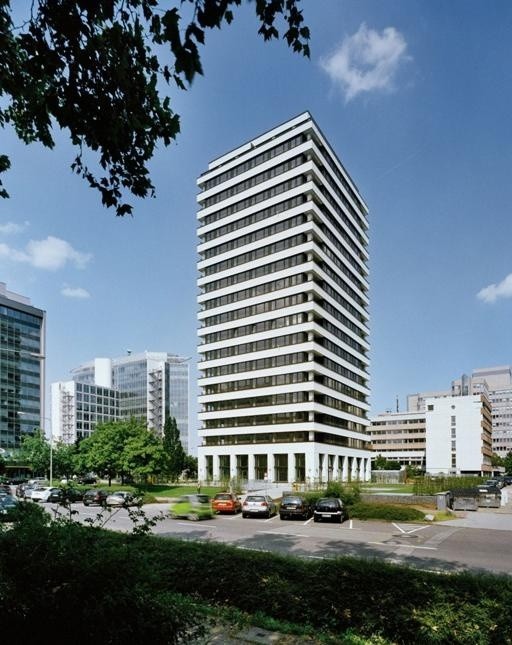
[437,491,454,510]
[292,483,301,492]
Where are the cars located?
[278,494,313,521]
[0,475,84,514]
[474,475,512,501]
[242,494,278,519]
[106,490,144,507]
[313,496,350,524]
[211,491,242,514]
[166,494,215,521]
[82,488,109,507]
